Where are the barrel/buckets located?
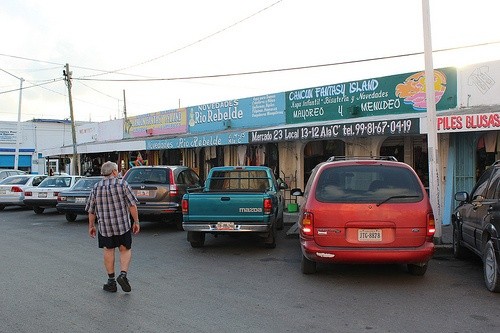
[287,203,298,213]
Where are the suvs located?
[289,155,436,275]
[450,159,500,292]
[121,165,205,231]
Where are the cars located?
[23,175,87,214]
[56,176,105,222]
[0,169,29,184]
[0,174,48,210]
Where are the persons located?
[84,160,140,293]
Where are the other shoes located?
[103,279,117,292]
[117,273,131,292]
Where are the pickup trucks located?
[180,165,289,251]
